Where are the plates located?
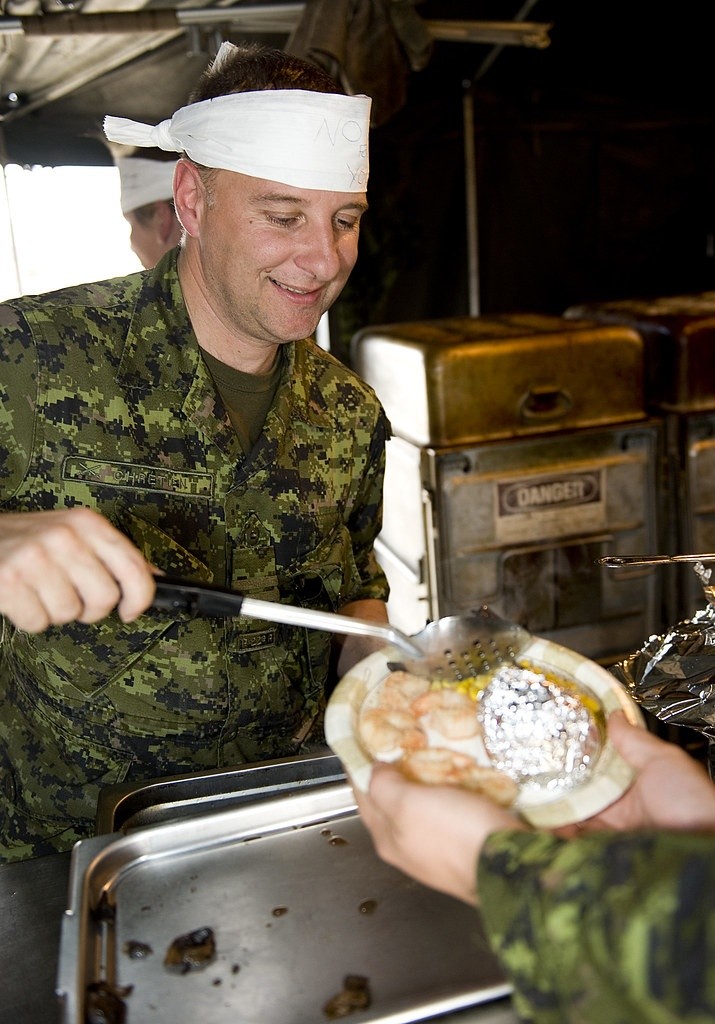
[324,631,647,833]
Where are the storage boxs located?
[351,296,715,664]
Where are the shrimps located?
[359,670,521,808]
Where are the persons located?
[118,139,185,271]
[0,48,392,865]
[353,710,715,1024]
